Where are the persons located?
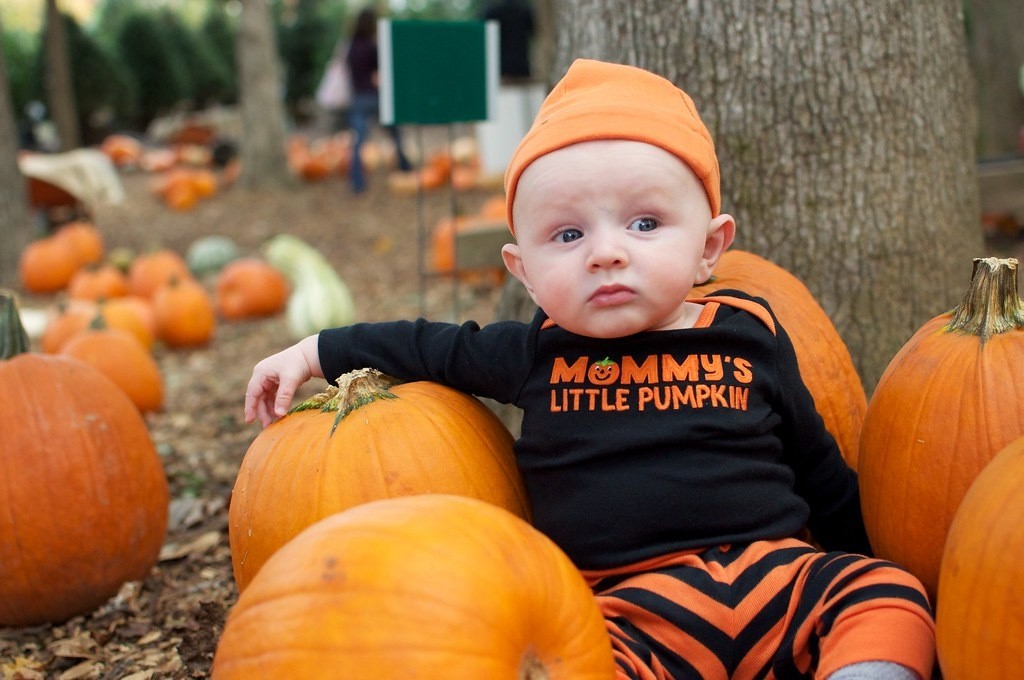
[243,60,938,679]
[348,6,411,192]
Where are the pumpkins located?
[0,130,1024,680]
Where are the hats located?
[504,58,721,239]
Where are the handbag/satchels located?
[315,36,355,111]
